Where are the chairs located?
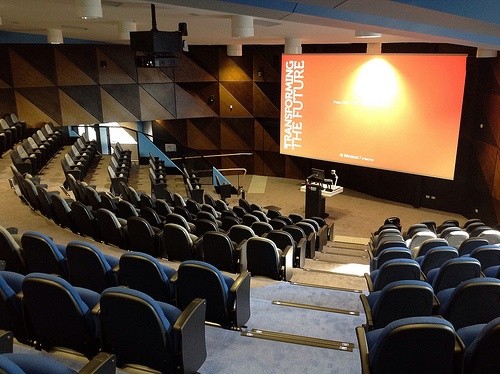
[0,113,334,279]
[355,218,500,374]
[0,225,252,374]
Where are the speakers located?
[179,23,188,36]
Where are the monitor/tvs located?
[311,167,325,181]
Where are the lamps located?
[476,47,497,58]
[285,36,304,55]
[76,0,103,20]
[227,45,243,57]
[47,29,65,44]
[354,30,381,38]
[367,44,382,54]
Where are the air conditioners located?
[230,15,255,38]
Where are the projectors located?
[134,54,178,68]
